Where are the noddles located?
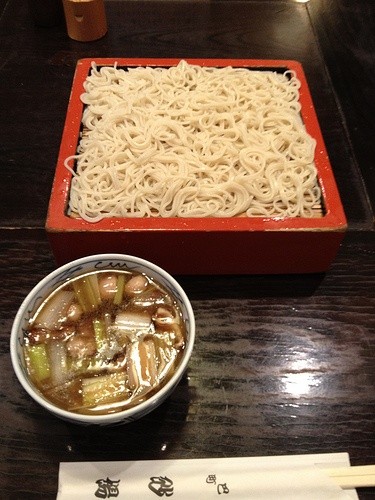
[64,58,323,224]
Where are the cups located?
[63,0,108,41]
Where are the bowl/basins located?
[45,57,349,280]
[10,254,195,427]
